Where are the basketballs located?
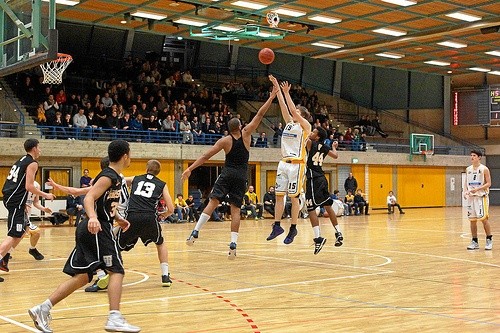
[258,48,275,65]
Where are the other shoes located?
[9,255,12,259]
[29,248,45,261]
[388,212,392,214]
[365,213,371,216]
[0,259,9,272]
[400,211,405,214]
[0,277,4,282]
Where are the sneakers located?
[466,240,480,249]
[28,304,54,333]
[97,274,109,289]
[265,225,284,241]
[83,279,108,292]
[334,231,343,247]
[161,273,173,287]
[228,242,236,261]
[186,230,199,246]
[484,237,494,250]
[283,227,298,244]
[103,314,141,332]
[312,237,327,255]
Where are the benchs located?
[329,111,452,155]
[59,209,76,226]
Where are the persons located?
[267,74,312,244]
[6,169,44,261]
[462,149,494,251]
[66,167,369,224]
[179,85,279,261]
[46,155,131,291]
[0,138,56,282]
[306,126,344,255]
[97,160,175,288]
[387,191,405,214]
[19,57,390,151]
[28,138,142,333]
